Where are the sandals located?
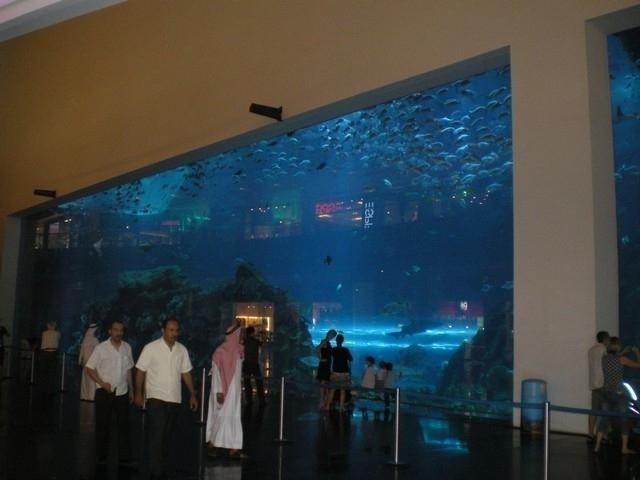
[585,434,615,446]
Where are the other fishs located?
[162,65,513,230]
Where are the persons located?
[584,329,613,442]
[314,329,338,412]
[592,334,640,455]
[383,363,404,403]
[0,325,11,383]
[374,360,387,401]
[324,333,353,414]
[39,318,63,365]
[204,323,250,461]
[77,322,101,404]
[134,316,199,480]
[242,326,269,411]
[359,355,376,400]
[82,319,135,480]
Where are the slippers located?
[204,447,250,459]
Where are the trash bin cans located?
[520,378,549,445]
